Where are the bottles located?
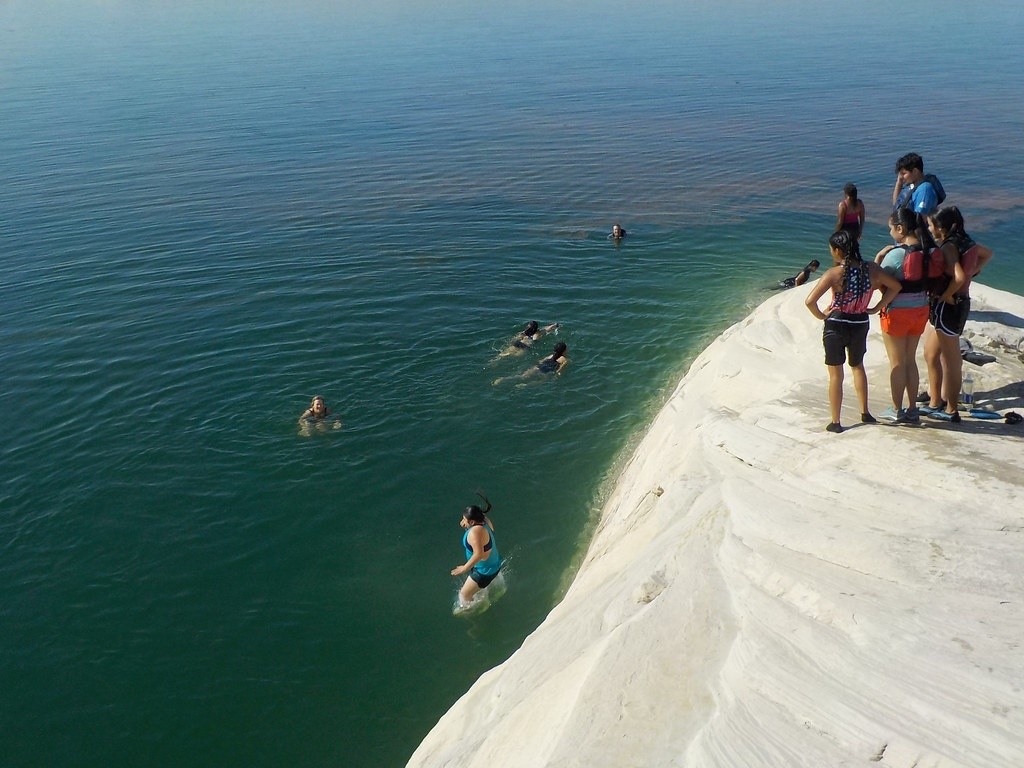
[961,373,973,411]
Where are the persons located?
[296,396,334,422]
[481,319,560,361]
[473,342,569,391]
[610,224,626,241]
[449,495,501,610]
[766,153,992,432]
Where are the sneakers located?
[917,404,943,416]
[877,406,904,421]
[927,410,961,422]
[902,407,920,422]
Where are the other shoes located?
[916,391,931,405]
[923,399,947,409]
